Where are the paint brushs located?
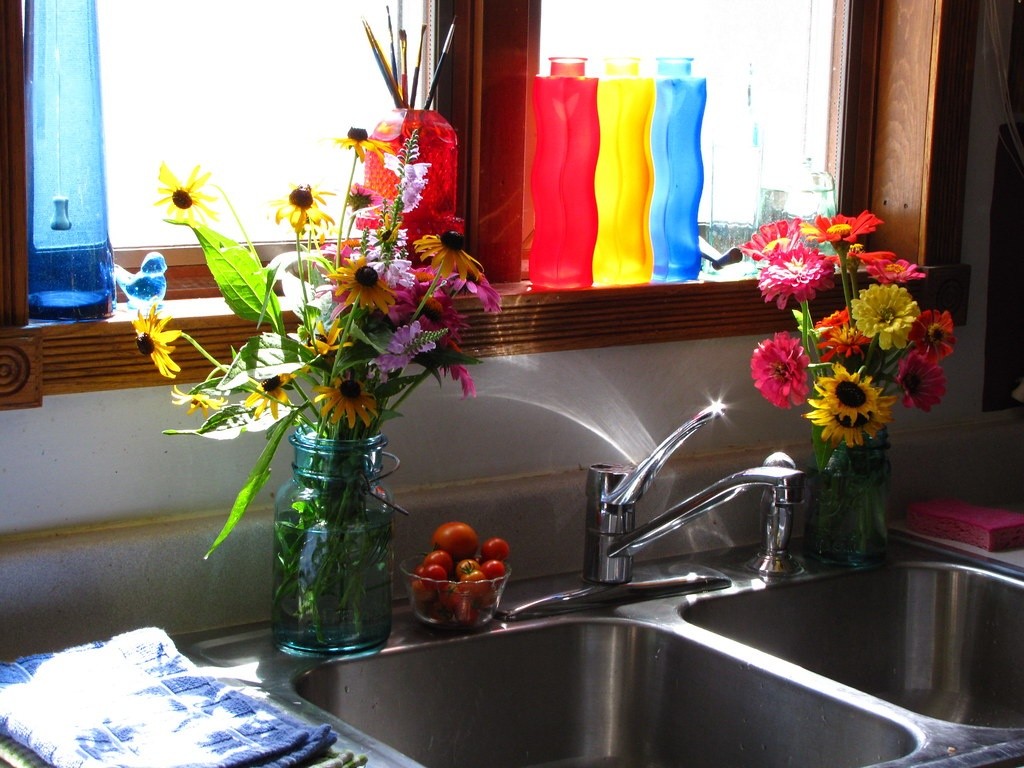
[364,5,458,121]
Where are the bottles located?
[21,0,115,322]
[592,56,657,292]
[526,55,600,292]
[651,57,709,284]
[364,109,462,284]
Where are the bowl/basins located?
[400,553,512,629]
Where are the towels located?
[0,704,370,768]
[1,620,338,768]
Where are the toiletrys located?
[747,159,843,270]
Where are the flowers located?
[739,211,960,561]
[128,128,502,650]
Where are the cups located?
[761,169,838,232]
[702,142,764,279]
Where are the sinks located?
[679,545,1024,750]
[197,603,945,768]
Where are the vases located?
[592,57,658,293]
[526,51,602,289]
[21,4,117,326]
[805,423,895,574]
[268,424,398,655]
[642,57,708,283]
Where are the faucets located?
[576,396,813,589]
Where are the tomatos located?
[409,523,511,628]
